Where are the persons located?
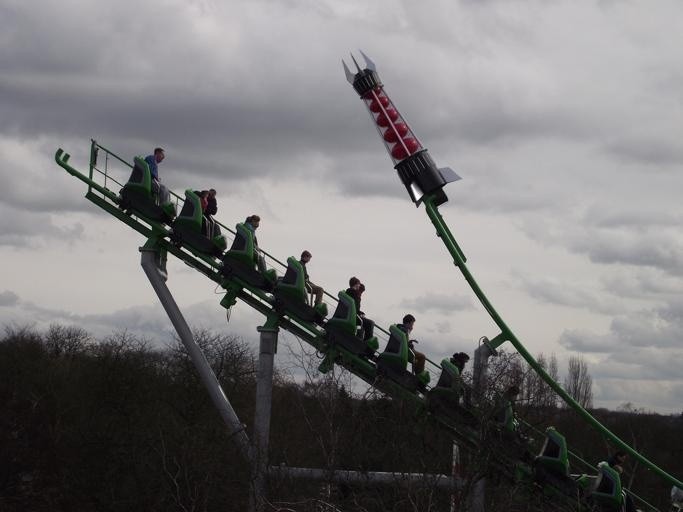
[346,277,374,340]
[495,386,535,445]
[397,314,426,375]
[144,147,169,204]
[300,250,324,305]
[609,451,636,511]
[451,352,479,409]
[193,188,221,239]
[244,215,266,270]
